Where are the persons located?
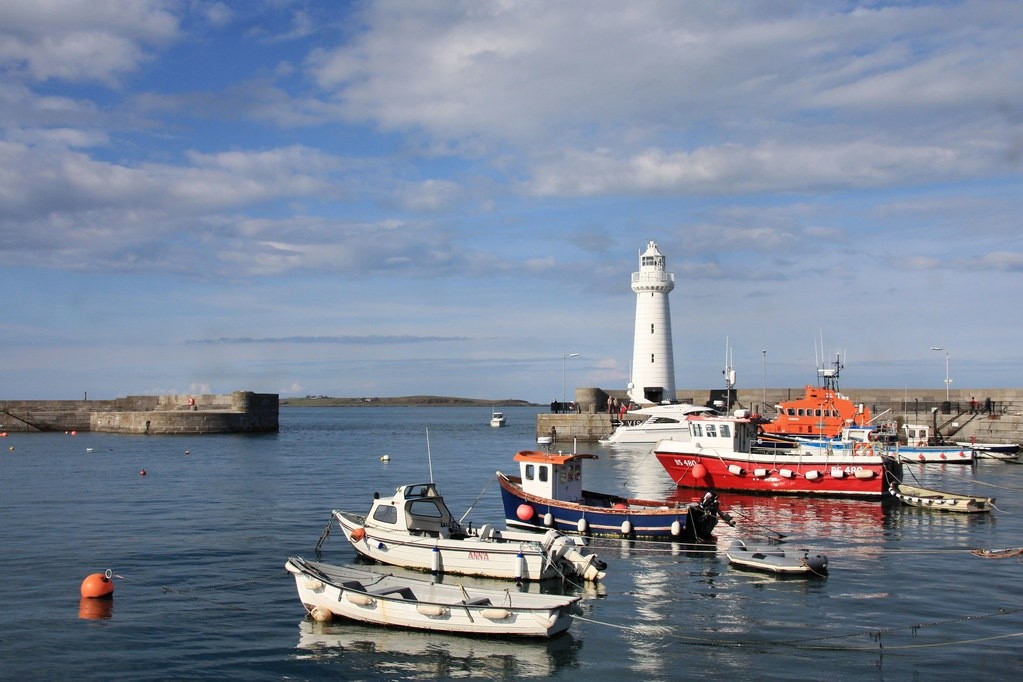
[607,396,613,414]
[613,398,619,413]
[986,397,991,414]
[970,397,978,415]
[620,404,627,413]
[553,398,558,414]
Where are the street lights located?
[762,349,767,411]
[931,346,949,401]
[562,353,579,414]
[489,412,506,427]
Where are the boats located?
[796,402,975,465]
[955,441,1021,459]
[727,545,829,574]
[331,424,611,582]
[651,334,905,500]
[496,448,737,544]
[888,479,996,514]
[284,555,585,639]
[754,329,873,445]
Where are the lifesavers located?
[852,443,873,456]
[916,441,927,446]
[868,430,879,442]
